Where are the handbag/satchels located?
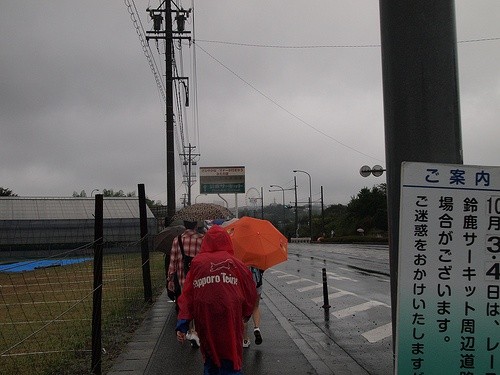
[183,255,195,279]
[166,272,180,303]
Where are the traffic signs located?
[199,166,245,195]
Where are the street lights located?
[269,184,290,239]
[292,170,316,237]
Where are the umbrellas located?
[168,202,235,223]
[357,228,364,232]
[204,220,229,228]
[224,216,288,270]
[153,225,186,255]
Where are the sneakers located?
[243,337,251,347]
[254,327,263,345]
[190,334,200,350]
[186,333,191,342]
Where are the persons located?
[242,266,265,348]
[174,223,259,375]
[165,220,214,353]
